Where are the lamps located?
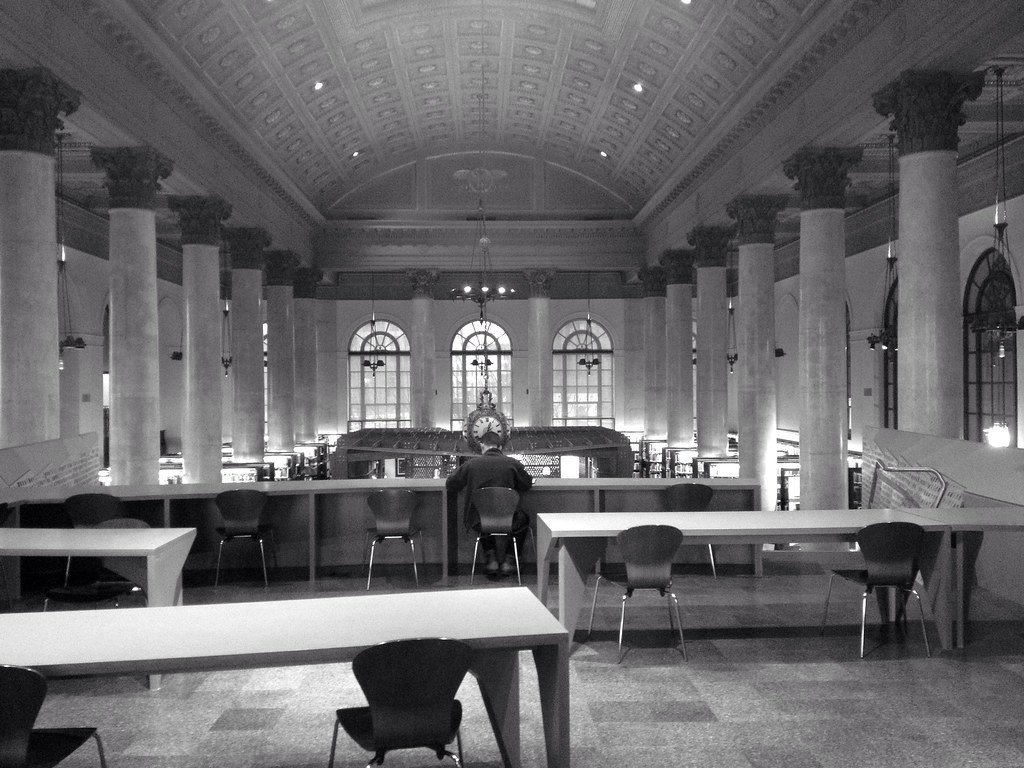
[449,95,516,382]
[866,69,1024,357]
[577,272,601,376]
[56,133,233,377]
[362,271,386,377]
[726,251,739,373]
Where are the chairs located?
[470,488,537,586]
[214,489,277,586]
[65,491,121,583]
[362,490,427,591]
[1,635,105,767]
[327,637,473,767]
[587,525,686,663]
[821,522,932,657]
[44,518,154,612]
[660,484,720,578]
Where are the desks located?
[1,479,1024,768]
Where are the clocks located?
[464,409,511,452]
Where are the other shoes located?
[483,562,499,573]
[502,563,521,575]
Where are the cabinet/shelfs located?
[620,429,862,511]
[100,433,343,485]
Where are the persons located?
[445,432,532,575]
